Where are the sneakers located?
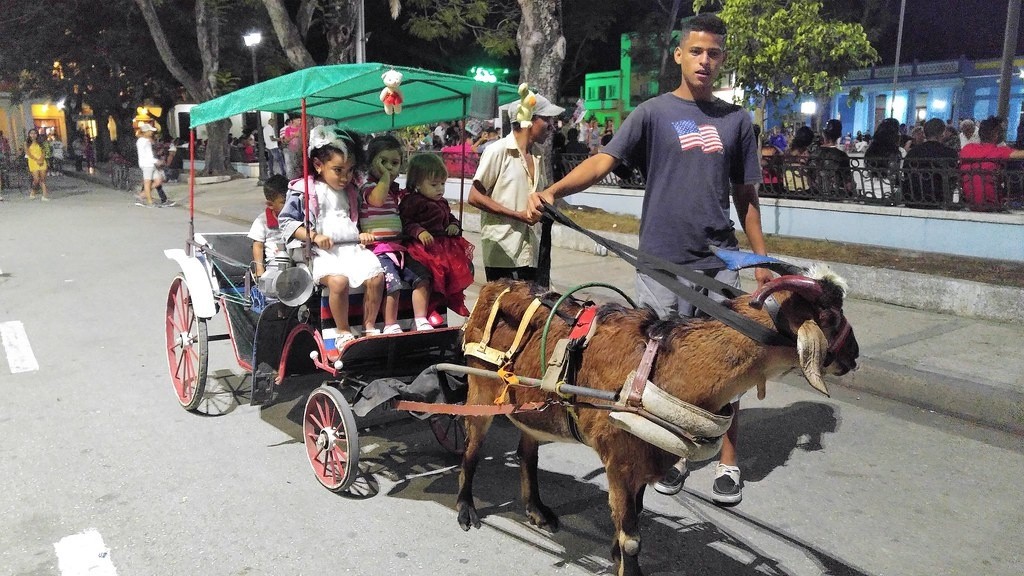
[653,461,688,495]
[712,462,742,504]
[410,316,434,331]
[383,324,403,333]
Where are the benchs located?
[193,232,315,370]
[298,259,448,362]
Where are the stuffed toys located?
[380,69,403,114]
[516,83,536,127]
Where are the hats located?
[823,119,842,136]
[508,94,566,124]
[141,124,157,132]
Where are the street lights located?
[242,27,271,186]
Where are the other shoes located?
[41,196,51,201]
[134,201,146,206]
[146,203,160,208]
[30,192,36,198]
[0,197,4,200]
[162,200,176,207]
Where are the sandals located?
[360,329,381,337]
[335,333,355,352]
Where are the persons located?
[0,127,260,201]
[262,118,286,177]
[280,117,304,179]
[525,15,776,505]
[134,105,177,207]
[357,110,1024,213]
[248,124,474,353]
[470,92,565,290]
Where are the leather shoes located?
[446,298,470,317]
[427,305,444,326]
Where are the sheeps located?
[454,261,861,576]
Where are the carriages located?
[164,62,860,576]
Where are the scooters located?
[111,151,132,191]
